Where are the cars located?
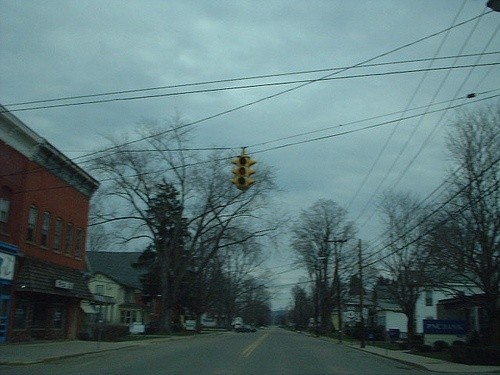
[235,325,257,333]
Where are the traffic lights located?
[231,149,257,192]
